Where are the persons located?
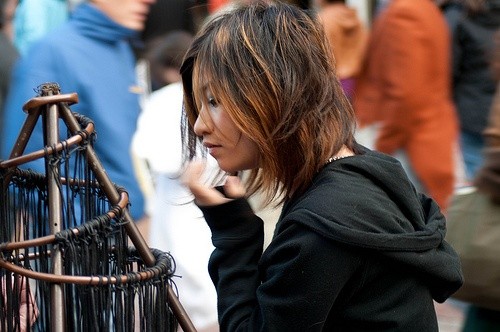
[168,1,440,332]
[0,0,156,248]
[0,0,500,332]
